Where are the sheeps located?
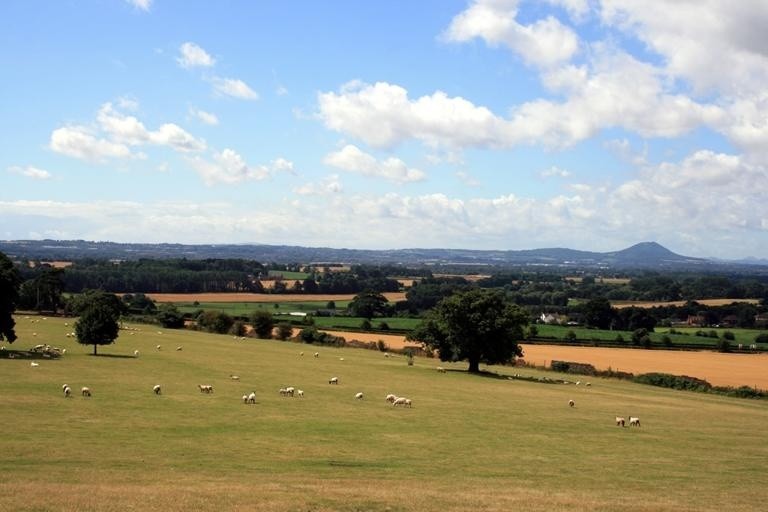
[198,384,213,393]
[436,366,446,374]
[153,385,161,395]
[229,375,240,382]
[328,377,338,385]
[354,393,363,400]
[543,377,591,387]
[242,391,256,404]
[279,386,304,398]
[614,415,641,428]
[82,388,91,397]
[62,383,72,398]
[568,400,575,407]
[385,395,411,408]
